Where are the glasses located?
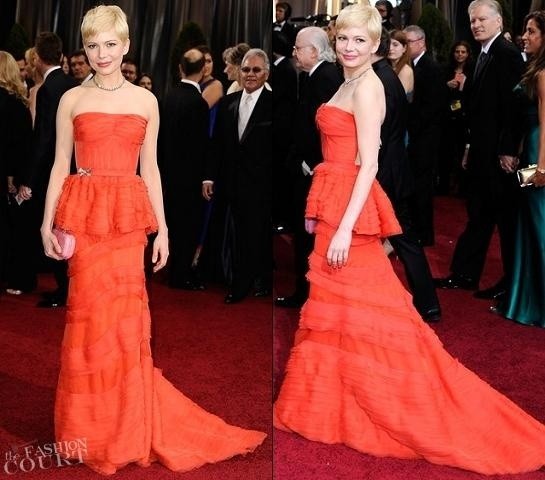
[241,66,261,73]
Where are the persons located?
[0,0,545,472]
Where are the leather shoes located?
[224,281,272,304]
[167,273,206,291]
[433,275,479,289]
[38,289,65,308]
[275,295,306,308]
[473,276,510,299]
[420,303,441,321]
